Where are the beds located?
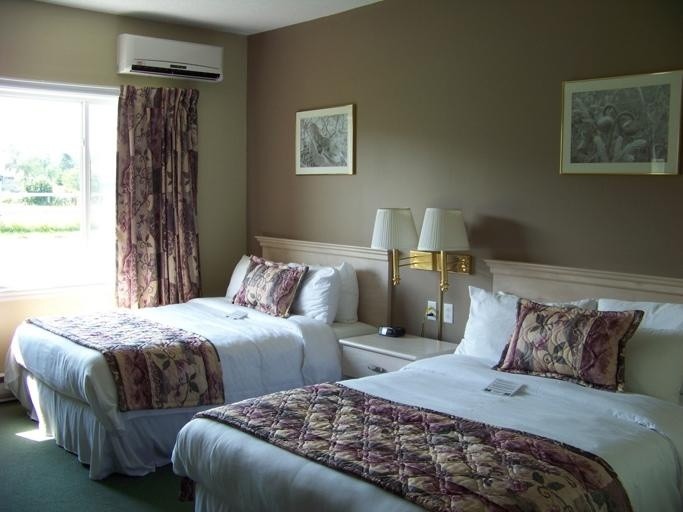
[169,258,683,511]
[2,233,394,483]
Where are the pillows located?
[230,253,310,318]
[225,254,251,304]
[595,297,683,409]
[333,259,361,324]
[490,296,645,396]
[290,266,337,326]
[453,283,597,368]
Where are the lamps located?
[370,207,435,286]
[416,206,474,295]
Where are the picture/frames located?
[293,102,356,176]
[558,68,683,176]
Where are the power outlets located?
[442,302,454,324]
[425,300,438,322]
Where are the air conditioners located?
[115,32,224,85]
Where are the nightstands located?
[338,330,459,377]
